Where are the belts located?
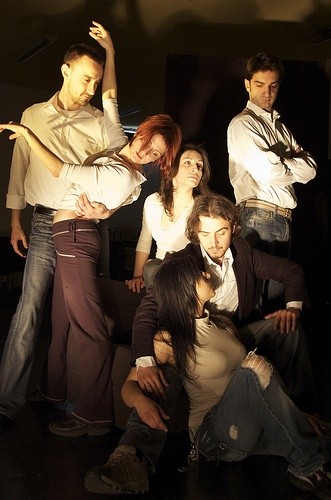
[34,206,58,216]
[240,200,293,220]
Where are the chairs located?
[96,275,146,428]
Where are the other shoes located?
[286,463,331,500]
[83,453,150,495]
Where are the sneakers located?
[27,384,64,404]
[48,412,113,438]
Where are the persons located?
[121,252,331,488]
[84,195,305,493]
[226,52,317,318]
[125,145,219,312]
[0,21,182,437]
[0,45,141,432]
